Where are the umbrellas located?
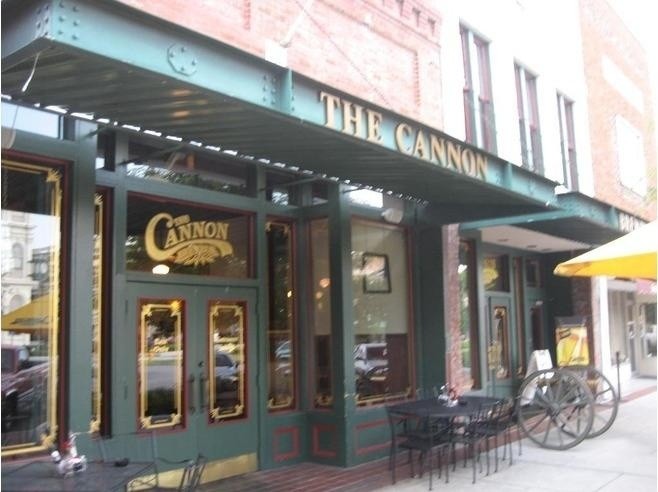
[553,220,657,281]
[1,295,50,331]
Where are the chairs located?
[386,395,523,491]
[124,457,208,492]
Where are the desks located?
[2,461,155,492]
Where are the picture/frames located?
[362,253,391,293]
